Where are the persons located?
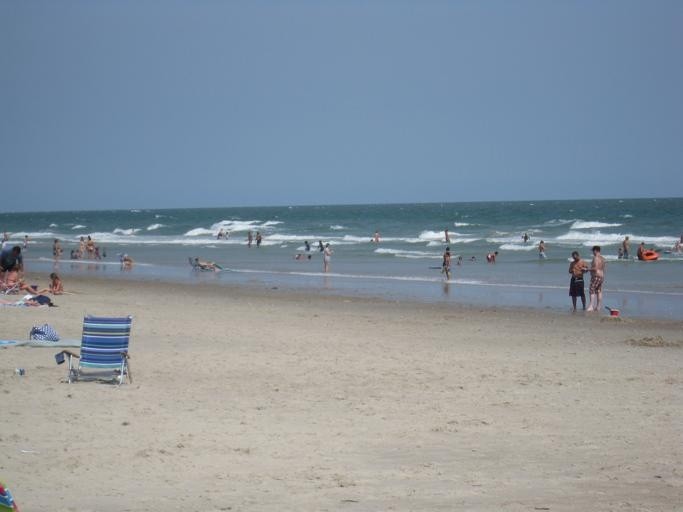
[582,246,608,312]
[188,226,335,275]
[374,230,381,242]
[437,228,683,282]
[0,229,137,307]
[566,250,588,310]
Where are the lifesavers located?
[640,249,659,259]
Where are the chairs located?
[55,315,134,389]
